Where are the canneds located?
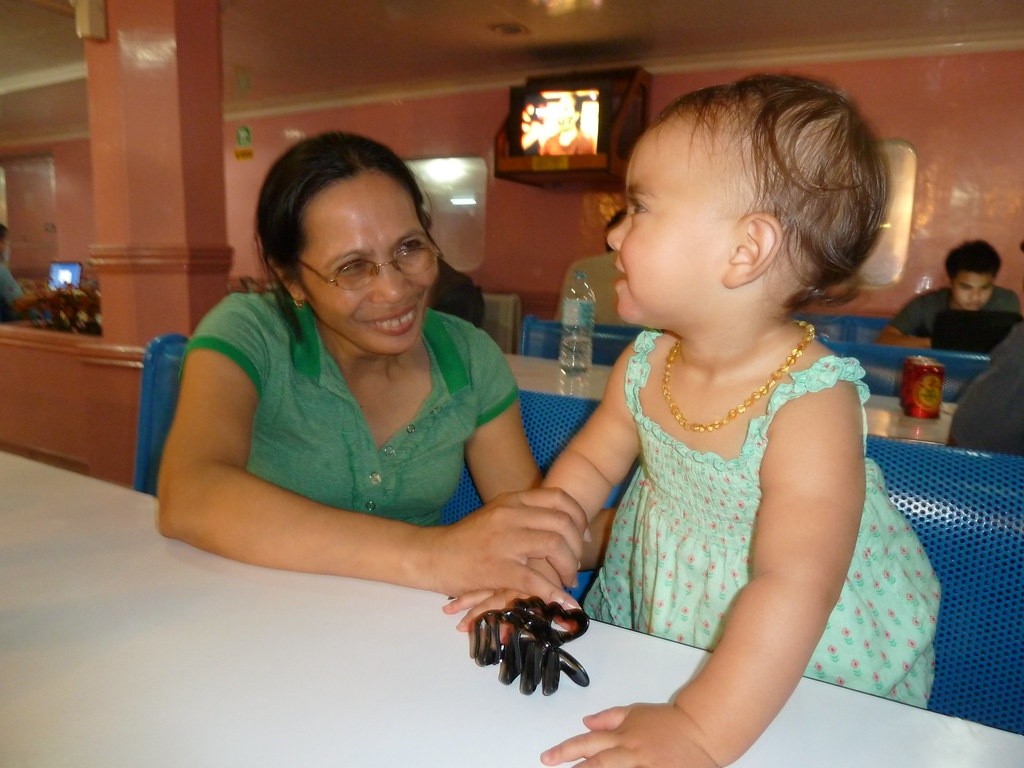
[901,356,945,419]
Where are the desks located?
[503,354,958,447]
[1,448,1024,766]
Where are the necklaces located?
[661,319,814,432]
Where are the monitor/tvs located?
[507,77,615,157]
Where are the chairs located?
[478,295,520,350]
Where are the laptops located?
[49,260,83,292]
[932,310,1022,353]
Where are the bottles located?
[558,272,596,375]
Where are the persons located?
[946,321,1024,458]
[541,94,596,155]
[556,210,651,328]
[0,224,52,322]
[444,72,945,768]
[876,239,1019,349]
[154,130,591,612]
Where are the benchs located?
[137,332,1024,738]
[516,314,990,408]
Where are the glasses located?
[292,227,447,290]
